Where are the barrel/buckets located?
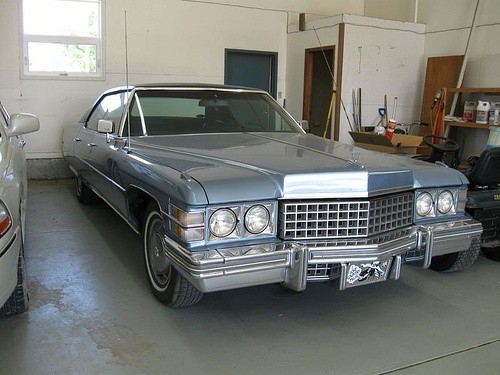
[464,101,476,121]
[476,100,490,124]
[488,102,500,125]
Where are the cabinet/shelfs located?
[442,89,500,137]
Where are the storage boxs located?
[349,132,431,154]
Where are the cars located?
[60,83,483,308]
[0,100,41,318]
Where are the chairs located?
[205,105,237,131]
[464,147,500,187]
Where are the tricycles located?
[389,133,500,248]
